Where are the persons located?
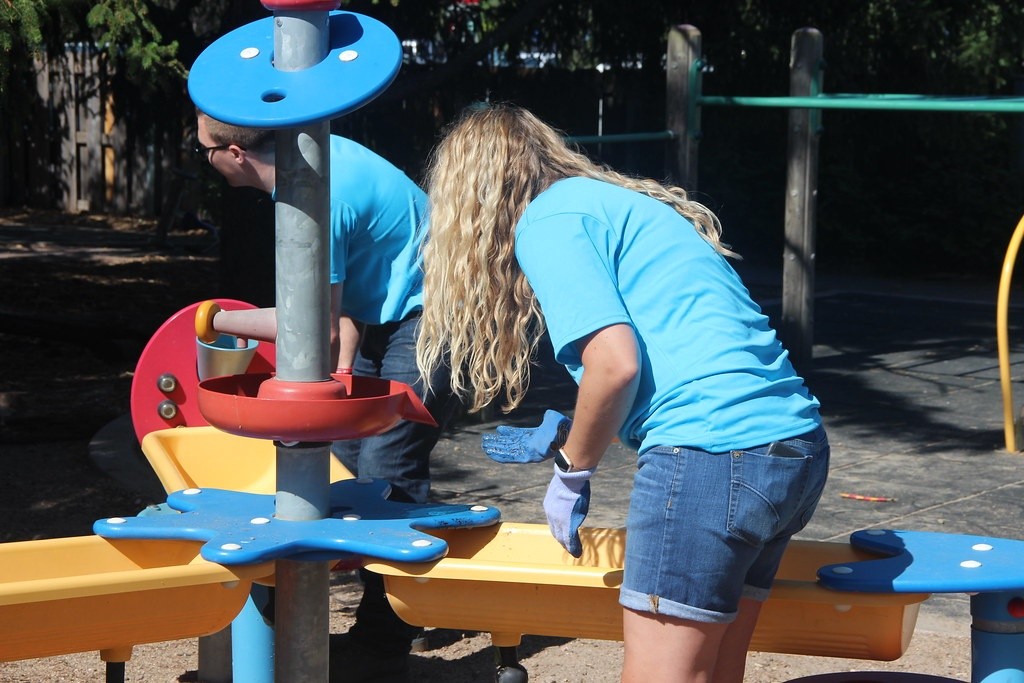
[415,106,831,683]
[196,110,457,682]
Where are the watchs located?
[555,447,589,474]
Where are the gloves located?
[542,464,597,558]
[482,410,572,464]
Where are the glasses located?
[192,139,248,160]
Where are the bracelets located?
[337,367,352,374]
